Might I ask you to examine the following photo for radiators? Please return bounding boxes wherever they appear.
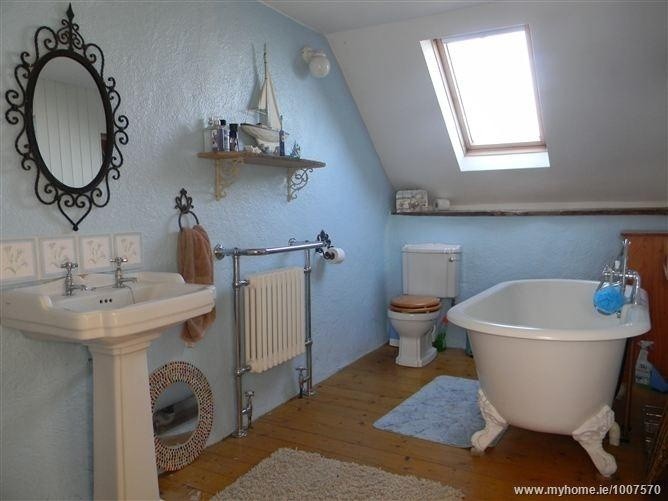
[246,267,303,372]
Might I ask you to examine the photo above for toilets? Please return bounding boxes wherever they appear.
[386,243,461,368]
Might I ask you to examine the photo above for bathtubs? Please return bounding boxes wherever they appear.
[447,279,651,476]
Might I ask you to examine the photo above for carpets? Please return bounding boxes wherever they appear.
[372,373,484,448]
[207,446,466,500]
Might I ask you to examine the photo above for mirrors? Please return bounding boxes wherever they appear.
[147,360,212,471]
[2,2,130,231]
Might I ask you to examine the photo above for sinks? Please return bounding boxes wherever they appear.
[1,269,217,347]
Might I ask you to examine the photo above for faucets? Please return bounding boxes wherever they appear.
[61,261,88,296]
[601,264,642,303]
[109,258,137,288]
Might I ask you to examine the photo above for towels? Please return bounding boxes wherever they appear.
[175,226,217,344]
